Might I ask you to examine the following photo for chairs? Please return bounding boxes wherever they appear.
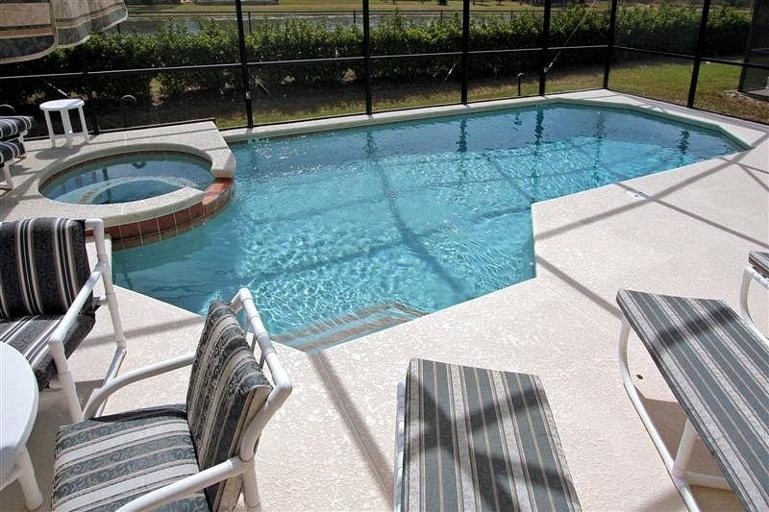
[0,215,129,424]
[0,104,34,191]
[49,286,293,512]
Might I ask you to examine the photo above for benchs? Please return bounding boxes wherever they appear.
[615,289,769,512]
[738,249,768,346]
[392,356,582,512]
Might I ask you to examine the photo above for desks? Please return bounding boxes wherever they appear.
[39,98,90,149]
[0,340,44,512]
